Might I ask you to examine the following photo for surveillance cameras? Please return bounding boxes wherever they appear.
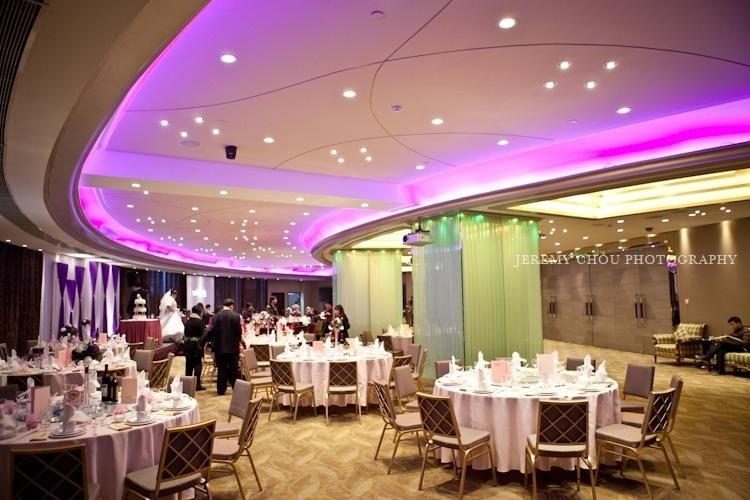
[225,145,237,159]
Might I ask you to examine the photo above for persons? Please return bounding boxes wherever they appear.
[184,304,207,391]
[158,288,185,344]
[695,316,750,376]
[265,296,278,322]
[242,302,256,324]
[285,300,350,346]
[203,304,215,325]
[125,279,149,318]
[198,299,242,395]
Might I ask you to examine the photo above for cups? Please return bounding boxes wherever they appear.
[386,323,413,336]
[449,356,603,394]
[284,346,387,361]
[251,314,307,328]
[248,329,296,344]
[147,313,159,319]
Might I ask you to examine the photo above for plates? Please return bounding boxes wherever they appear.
[2,329,124,373]
[1,388,192,439]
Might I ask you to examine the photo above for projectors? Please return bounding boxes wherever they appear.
[403,233,434,245]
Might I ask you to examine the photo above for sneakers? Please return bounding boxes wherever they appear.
[196,386,206,391]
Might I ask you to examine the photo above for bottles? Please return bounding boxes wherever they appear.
[99,361,118,403]
